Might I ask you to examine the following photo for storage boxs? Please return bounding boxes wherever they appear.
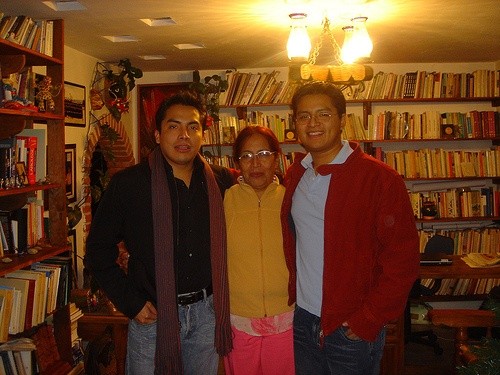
[68,290,93,313]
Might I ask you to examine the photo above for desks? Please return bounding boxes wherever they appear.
[381,254,500,375]
[77,309,130,375]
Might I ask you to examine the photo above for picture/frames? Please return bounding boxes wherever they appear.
[44,144,77,210]
[34,72,87,129]
[68,229,78,288]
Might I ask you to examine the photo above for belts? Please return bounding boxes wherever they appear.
[177,289,211,305]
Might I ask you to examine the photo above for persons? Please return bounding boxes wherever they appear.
[216,125,295,375]
[215,81,420,375]
[84,93,227,375]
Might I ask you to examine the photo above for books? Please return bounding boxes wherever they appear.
[342,110,499,137]
[2,62,36,105]
[201,112,295,145]
[360,69,499,97]
[360,142,500,178]
[206,148,294,175]
[0,257,85,375]
[417,226,500,269]
[5,123,48,185]
[415,277,500,296]
[411,299,483,324]
[0,12,54,57]
[225,70,296,106]
[0,200,43,262]
[408,183,500,218]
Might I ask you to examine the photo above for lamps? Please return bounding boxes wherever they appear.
[340,16,374,63]
[287,13,312,65]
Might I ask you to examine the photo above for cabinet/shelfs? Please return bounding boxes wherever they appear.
[201,97,500,301]
[0,17,77,375]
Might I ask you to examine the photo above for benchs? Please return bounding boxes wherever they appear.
[426,308,500,375]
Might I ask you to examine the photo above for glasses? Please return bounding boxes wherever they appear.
[238,150,275,161]
[295,111,337,125]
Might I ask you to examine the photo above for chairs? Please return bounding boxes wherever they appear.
[404,235,455,356]
